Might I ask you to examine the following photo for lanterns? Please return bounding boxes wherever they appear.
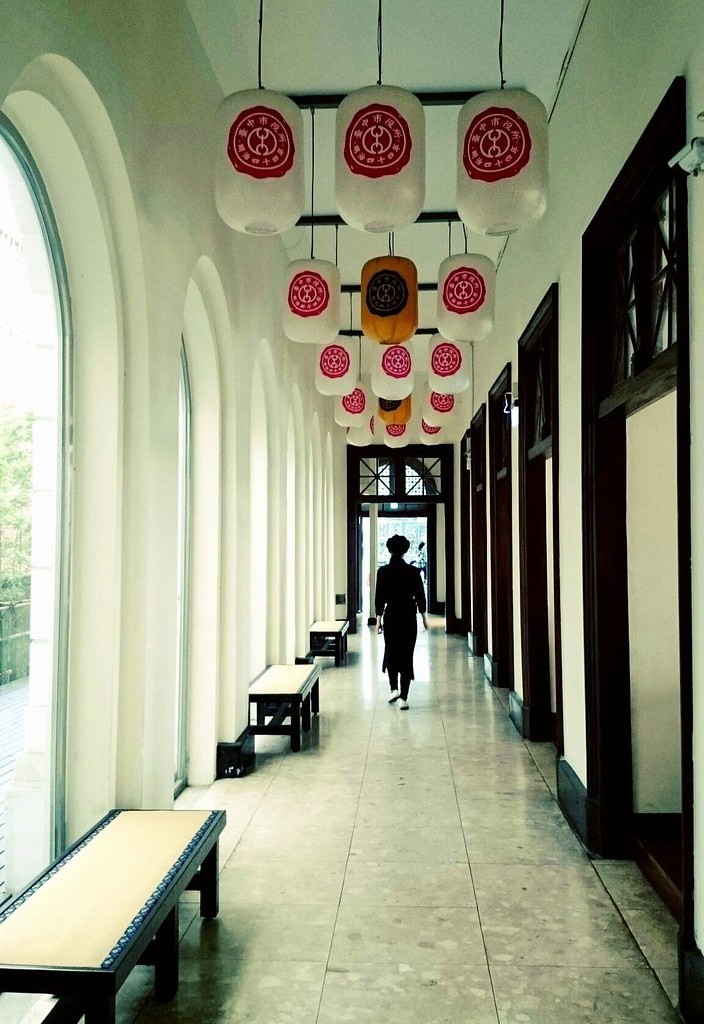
[372,340,413,400]
[360,255,418,345]
[437,253,496,342]
[429,334,470,394]
[315,335,356,395]
[455,88,550,237]
[282,259,341,344]
[334,85,426,234]
[212,89,304,236]
[333,381,456,448]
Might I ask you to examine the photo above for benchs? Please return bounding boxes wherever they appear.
[309,620,349,667]
[0,809,227,1024]
[249,664,322,751]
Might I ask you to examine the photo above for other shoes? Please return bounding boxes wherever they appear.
[397,698,412,710]
[387,689,401,703]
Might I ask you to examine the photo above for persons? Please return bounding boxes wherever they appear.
[416,542,427,583]
[375,534,428,710]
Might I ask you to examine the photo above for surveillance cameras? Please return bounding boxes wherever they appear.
[668,136,704,173]
